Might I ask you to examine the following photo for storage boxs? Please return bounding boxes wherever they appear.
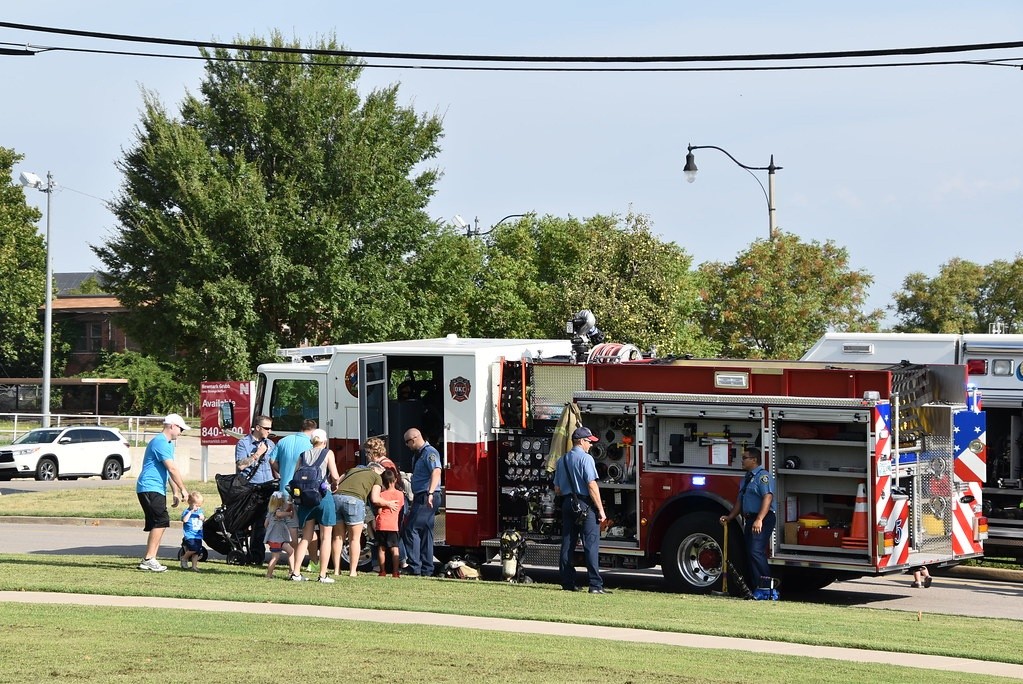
[785,522,797,545]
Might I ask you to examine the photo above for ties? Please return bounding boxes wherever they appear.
[739,474,753,526]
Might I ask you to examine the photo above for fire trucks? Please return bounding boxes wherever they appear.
[797,332,1023,572]
[251,308,988,599]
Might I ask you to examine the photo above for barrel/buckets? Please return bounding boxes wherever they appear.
[799,512,827,527]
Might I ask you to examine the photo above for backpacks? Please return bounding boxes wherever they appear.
[285,449,330,506]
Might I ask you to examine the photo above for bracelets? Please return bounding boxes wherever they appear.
[253,455,257,460]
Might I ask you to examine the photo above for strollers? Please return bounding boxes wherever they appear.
[178,473,256,565]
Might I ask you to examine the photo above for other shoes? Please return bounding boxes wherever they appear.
[393,571,400,578]
[911,581,921,588]
[180,556,188,569]
[378,571,386,576]
[924,576,932,588]
[401,566,420,576]
[191,567,199,572]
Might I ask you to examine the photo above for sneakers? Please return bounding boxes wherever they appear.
[317,575,335,582]
[140,557,167,572]
[291,573,310,581]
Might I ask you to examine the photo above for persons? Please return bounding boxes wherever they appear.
[331,462,400,577]
[911,565,932,588]
[404,427,442,576]
[375,469,405,578]
[268,419,319,573]
[552,426,613,595]
[286,429,340,583]
[366,503,379,546]
[136,413,190,572]
[719,447,777,600]
[263,491,295,581]
[235,416,280,566]
[181,491,205,572]
[364,437,409,571]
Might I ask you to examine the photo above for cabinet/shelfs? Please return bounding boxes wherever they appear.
[775,438,867,478]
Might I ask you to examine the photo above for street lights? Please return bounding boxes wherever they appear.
[682,145,776,246]
[20,172,52,428]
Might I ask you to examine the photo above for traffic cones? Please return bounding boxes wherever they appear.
[843,483,869,551]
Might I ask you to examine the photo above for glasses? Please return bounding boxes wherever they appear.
[405,437,417,444]
[256,423,272,430]
[179,427,183,433]
[742,456,754,460]
[583,438,592,444]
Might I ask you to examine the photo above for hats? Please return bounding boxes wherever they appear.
[311,429,327,441]
[163,414,191,430]
[572,427,599,441]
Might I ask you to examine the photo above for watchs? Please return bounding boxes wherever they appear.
[427,492,433,496]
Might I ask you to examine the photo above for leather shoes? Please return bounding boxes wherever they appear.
[562,587,588,593]
[588,589,613,594]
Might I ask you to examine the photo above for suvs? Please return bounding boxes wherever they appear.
[0,423,131,480]
